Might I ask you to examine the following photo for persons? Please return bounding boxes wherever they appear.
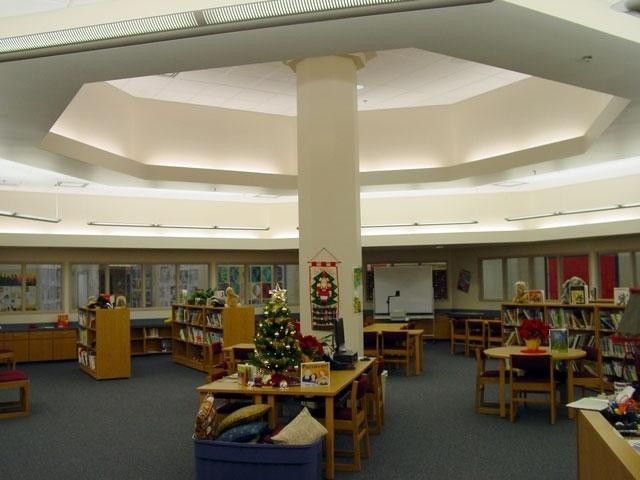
[302,368,329,386]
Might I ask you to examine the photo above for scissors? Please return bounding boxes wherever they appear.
[619,395,635,408]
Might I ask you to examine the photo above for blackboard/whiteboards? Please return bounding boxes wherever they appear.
[364,267,448,302]
[373,266,433,314]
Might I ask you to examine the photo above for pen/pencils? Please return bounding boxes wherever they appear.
[607,400,626,416]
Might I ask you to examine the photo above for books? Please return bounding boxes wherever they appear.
[76,308,96,373]
[501,286,639,394]
[171,307,224,345]
[300,361,331,389]
[549,328,569,354]
[564,397,610,411]
[237,364,261,387]
[149,326,171,352]
